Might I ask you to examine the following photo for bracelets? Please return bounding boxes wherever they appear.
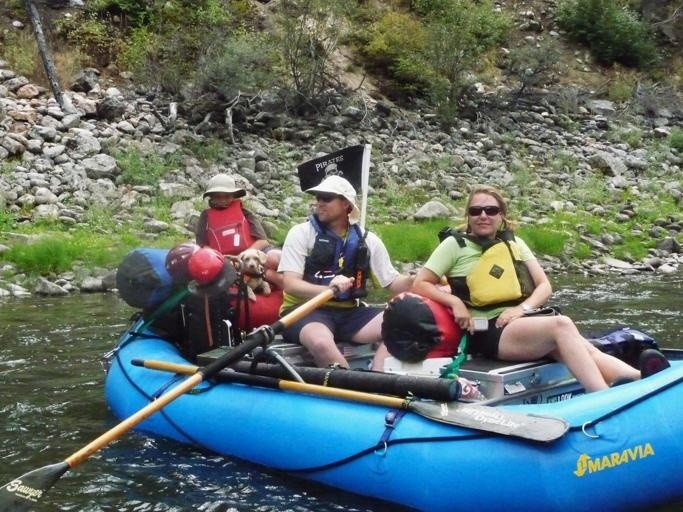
[520,302,531,312]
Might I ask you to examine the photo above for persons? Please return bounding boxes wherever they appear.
[411,184,670,394]
[277,175,417,373]
[196,173,284,289]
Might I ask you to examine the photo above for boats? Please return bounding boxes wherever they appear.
[101,312,683,512]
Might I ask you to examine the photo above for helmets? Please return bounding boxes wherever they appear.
[187,246,225,286]
[165,243,200,281]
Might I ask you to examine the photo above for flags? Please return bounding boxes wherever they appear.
[298,143,372,233]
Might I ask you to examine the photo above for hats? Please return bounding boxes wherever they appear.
[304,175,361,226]
[203,173,246,200]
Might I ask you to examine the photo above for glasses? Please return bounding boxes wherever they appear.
[316,192,345,202]
[468,206,502,216]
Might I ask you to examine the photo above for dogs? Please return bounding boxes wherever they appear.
[238,249,272,302]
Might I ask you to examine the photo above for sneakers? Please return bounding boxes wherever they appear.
[637,348,671,380]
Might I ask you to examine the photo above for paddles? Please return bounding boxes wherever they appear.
[127,357,569,448]
[0,270,355,506]
